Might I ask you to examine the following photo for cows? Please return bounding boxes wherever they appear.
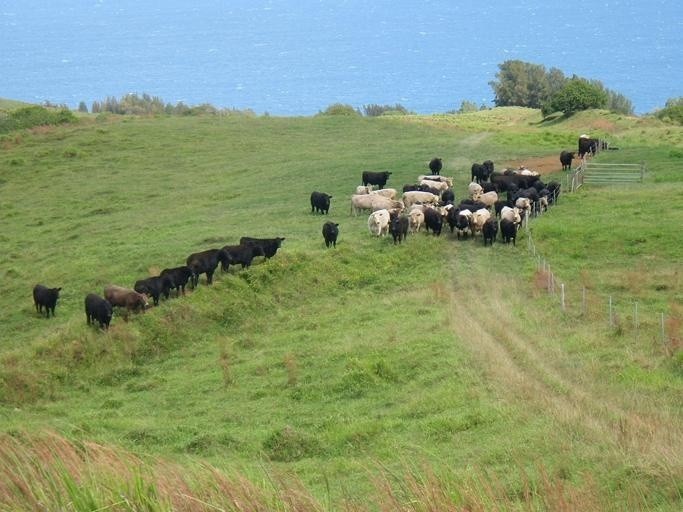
[32,284,62,319]
[310,191,332,215]
[347,157,562,247]
[322,220,340,249]
[557,132,604,170]
[84,235,286,329]
